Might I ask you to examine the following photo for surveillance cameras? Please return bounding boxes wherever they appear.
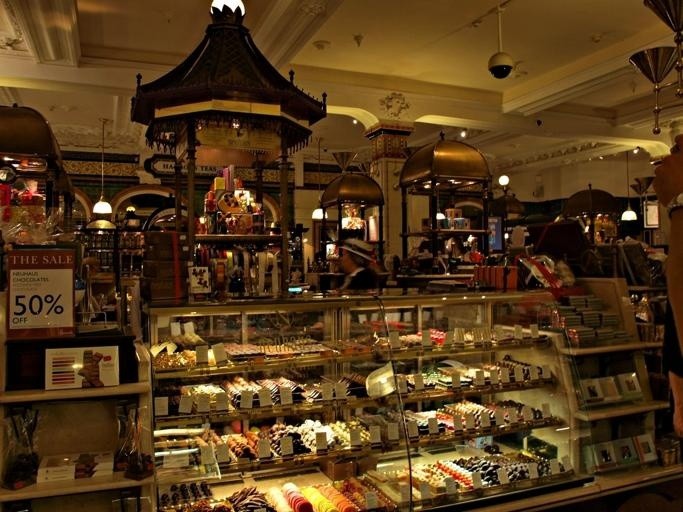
[488,53,516,80]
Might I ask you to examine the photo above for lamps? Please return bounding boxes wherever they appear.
[620,152,637,220]
[92,118,112,213]
[629,0,683,135]
[311,136,327,219]
[629,176,659,215]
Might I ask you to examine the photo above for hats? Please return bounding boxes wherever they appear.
[336,238,376,262]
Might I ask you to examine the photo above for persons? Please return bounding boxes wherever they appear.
[330,239,387,290]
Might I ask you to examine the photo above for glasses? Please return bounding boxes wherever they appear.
[338,251,350,258]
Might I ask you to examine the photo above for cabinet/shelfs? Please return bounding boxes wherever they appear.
[493,278,683,496]
[142,295,410,511]
[338,293,600,512]
[0,329,156,512]
